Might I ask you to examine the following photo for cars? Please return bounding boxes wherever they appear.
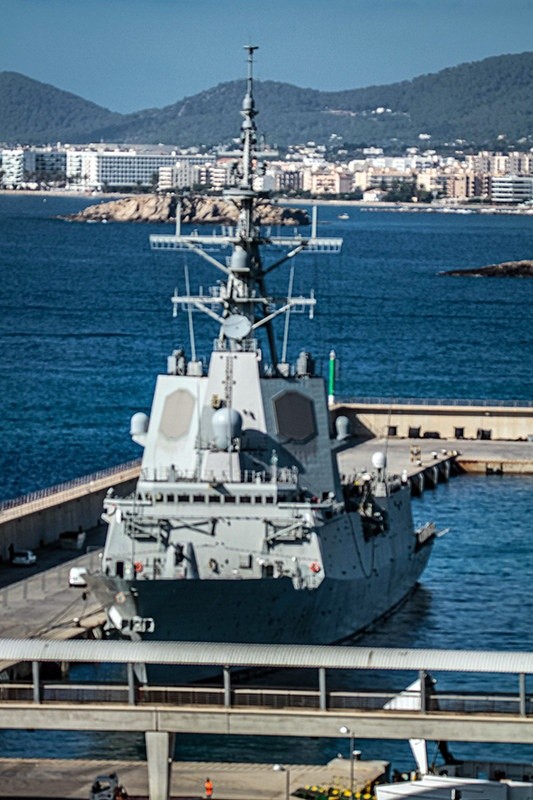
[11,550,38,567]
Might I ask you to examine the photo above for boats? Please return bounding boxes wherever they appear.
[66,46,436,685]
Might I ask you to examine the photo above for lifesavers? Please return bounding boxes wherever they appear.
[310,562,320,572]
[134,562,143,573]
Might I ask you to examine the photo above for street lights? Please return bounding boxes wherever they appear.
[273,763,290,800]
[338,725,354,799]
